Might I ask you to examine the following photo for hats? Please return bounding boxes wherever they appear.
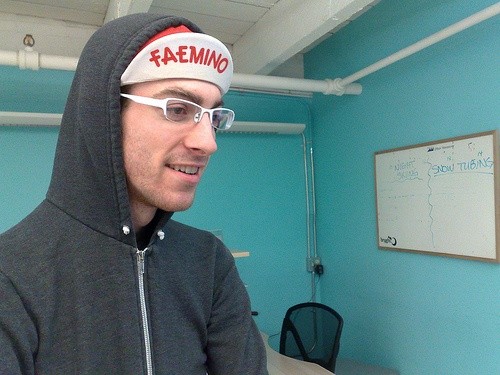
[119,25,233,96]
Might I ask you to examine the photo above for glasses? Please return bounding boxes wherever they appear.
[120,93,235,130]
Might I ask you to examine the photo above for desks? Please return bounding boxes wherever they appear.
[260,331,338,375]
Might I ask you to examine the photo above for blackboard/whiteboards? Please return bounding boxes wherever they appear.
[373,129,500,263]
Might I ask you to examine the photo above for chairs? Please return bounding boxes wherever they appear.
[278,302,345,374]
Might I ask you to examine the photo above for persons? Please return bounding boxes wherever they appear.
[0,13,271,375]
